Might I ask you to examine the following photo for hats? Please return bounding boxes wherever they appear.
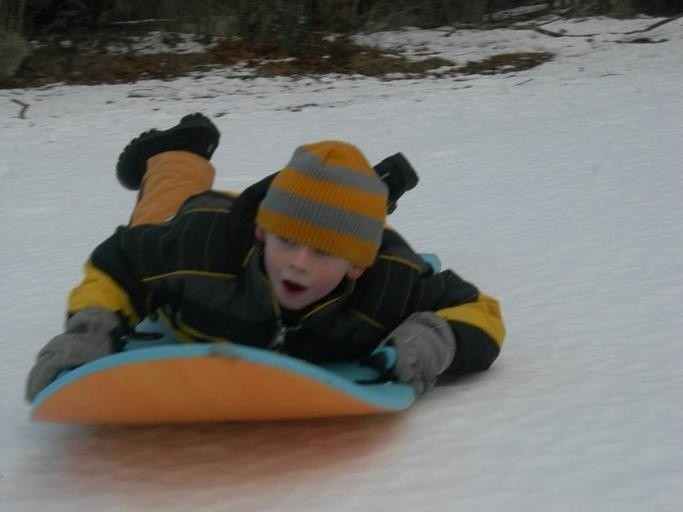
[254,139,389,268]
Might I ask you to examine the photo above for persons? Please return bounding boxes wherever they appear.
[26,112,506,404]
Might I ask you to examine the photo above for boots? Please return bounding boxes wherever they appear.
[115,113,221,191]
[373,153,419,215]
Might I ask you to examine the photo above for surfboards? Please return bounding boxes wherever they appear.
[29,253,442,423]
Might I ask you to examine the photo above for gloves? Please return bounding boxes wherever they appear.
[381,310,457,393]
[25,308,122,402]
